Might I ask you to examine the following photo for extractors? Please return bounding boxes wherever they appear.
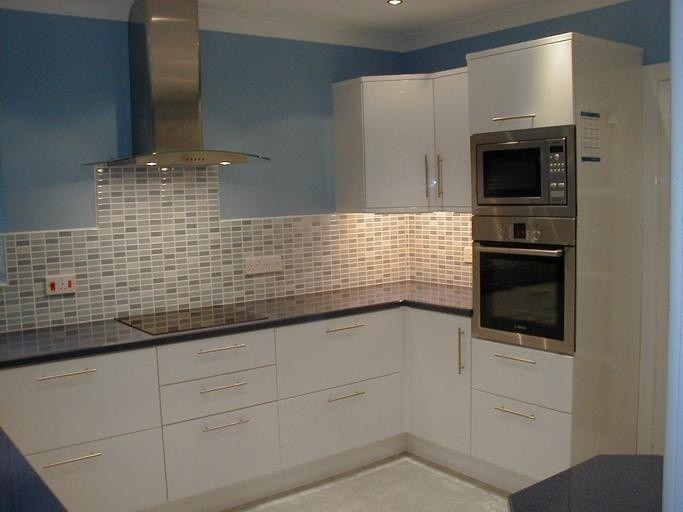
[80,0,272,168]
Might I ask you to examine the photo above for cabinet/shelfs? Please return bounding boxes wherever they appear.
[157,327,280,510]
[276,307,406,477]
[465,32,575,132]
[1,343,167,512]
[329,64,472,212]
[470,338,573,493]
[403,304,474,479]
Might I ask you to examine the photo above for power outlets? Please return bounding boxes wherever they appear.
[44,272,75,296]
[243,254,283,276]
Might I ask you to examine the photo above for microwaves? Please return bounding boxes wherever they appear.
[468,125,577,204]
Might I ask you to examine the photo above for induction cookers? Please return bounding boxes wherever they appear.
[114,304,268,336]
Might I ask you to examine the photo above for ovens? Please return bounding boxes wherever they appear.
[473,218,574,357]
